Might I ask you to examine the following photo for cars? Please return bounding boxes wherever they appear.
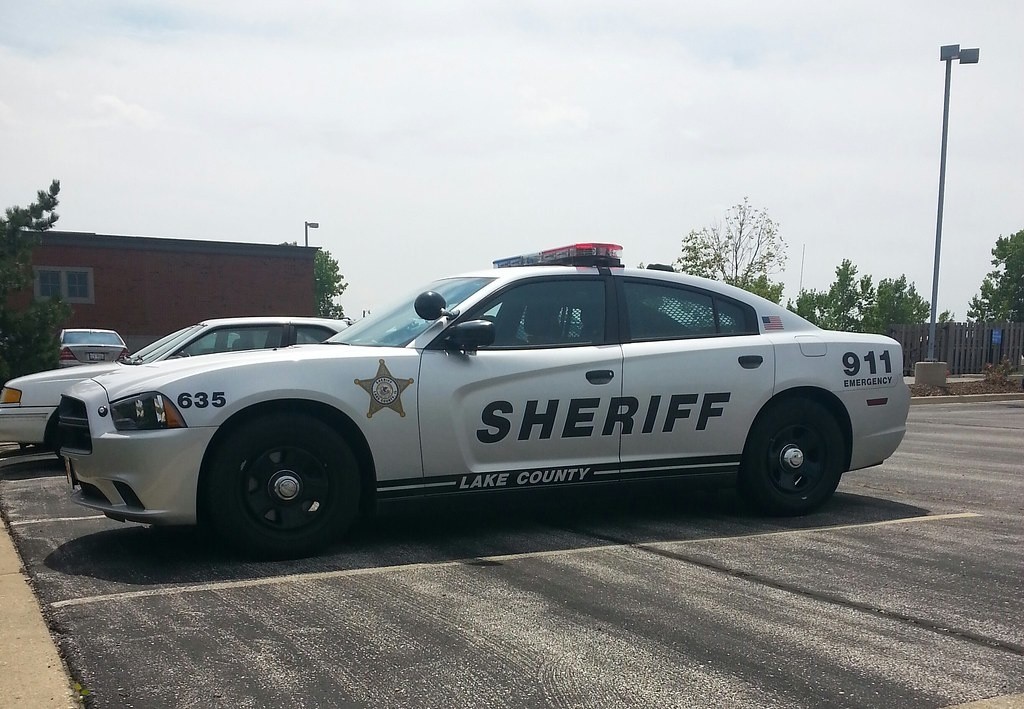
[0,317,349,468]
[59,243,911,563]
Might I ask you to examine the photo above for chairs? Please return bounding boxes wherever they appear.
[578,302,607,342]
[231,338,256,351]
[523,299,565,343]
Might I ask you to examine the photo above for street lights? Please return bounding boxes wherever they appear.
[305,221,319,247]
[913,44,979,382]
